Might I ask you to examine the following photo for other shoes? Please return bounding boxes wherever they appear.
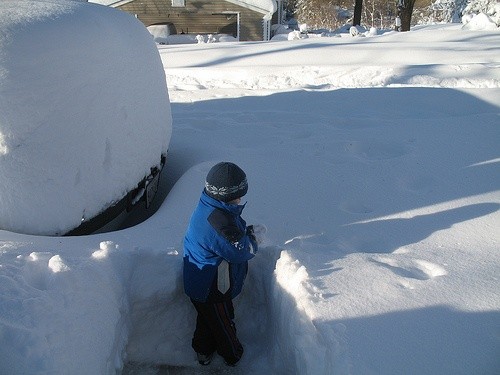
[196,352,211,365]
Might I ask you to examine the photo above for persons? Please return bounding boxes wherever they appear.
[182,162,267,367]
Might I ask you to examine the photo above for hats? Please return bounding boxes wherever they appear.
[204,162,248,201]
